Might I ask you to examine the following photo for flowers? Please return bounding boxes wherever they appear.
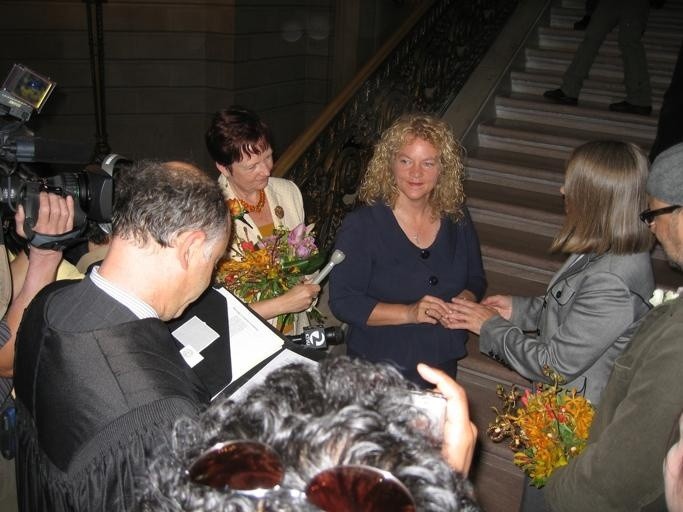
[217,222,317,327]
[486,369,598,487]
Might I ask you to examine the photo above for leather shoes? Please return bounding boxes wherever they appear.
[609,101,654,115]
[540,88,577,106]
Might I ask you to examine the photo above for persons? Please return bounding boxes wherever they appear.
[1,104,321,511]
[329,113,488,382]
[443,143,683,511]
[542,1,653,116]
[138,364,477,512]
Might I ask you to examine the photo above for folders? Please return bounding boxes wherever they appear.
[165,283,329,404]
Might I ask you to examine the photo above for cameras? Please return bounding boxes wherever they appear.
[407,388,449,440]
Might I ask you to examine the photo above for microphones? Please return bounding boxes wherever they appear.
[290,325,342,351]
[311,249,346,286]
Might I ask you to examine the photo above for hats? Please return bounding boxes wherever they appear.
[643,143,682,204]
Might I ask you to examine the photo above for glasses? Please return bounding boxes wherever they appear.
[181,438,417,512]
[633,204,683,224]
